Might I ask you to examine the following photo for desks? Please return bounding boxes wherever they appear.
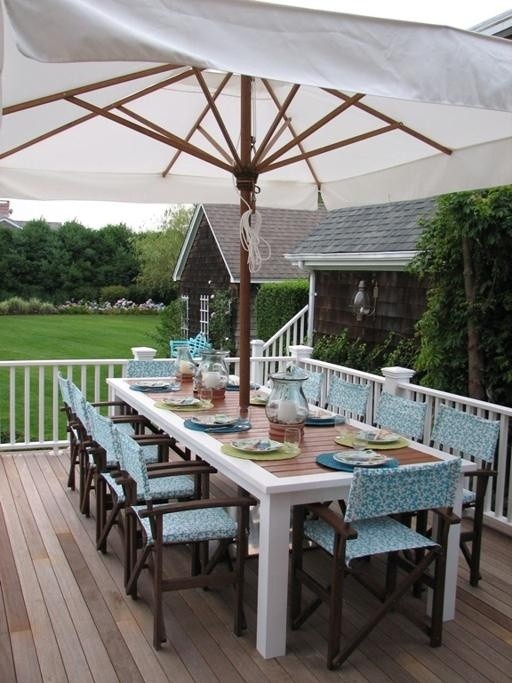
[103,374,478,658]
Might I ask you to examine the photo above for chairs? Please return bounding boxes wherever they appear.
[117,429,256,649]
[86,403,216,600]
[56,375,152,488]
[290,459,466,670]
[126,362,501,586]
[69,383,192,516]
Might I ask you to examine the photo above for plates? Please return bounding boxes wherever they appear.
[229,379,239,386]
[230,436,284,453]
[331,449,391,468]
[162,396,199,406]
[256,395,267,401]
[135,379,167,388]
[307,407,335,420]
[190,413,237,426]
[359,429,400,442]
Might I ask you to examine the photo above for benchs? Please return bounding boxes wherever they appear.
[170,335,213,358]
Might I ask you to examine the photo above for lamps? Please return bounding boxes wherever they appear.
[346,278,380,323]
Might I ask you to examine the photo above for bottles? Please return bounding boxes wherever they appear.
[174,346,197,375]
[264,371,309,424]
[192,349,231,390]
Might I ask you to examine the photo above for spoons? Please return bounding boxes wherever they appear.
[206,424,253,433]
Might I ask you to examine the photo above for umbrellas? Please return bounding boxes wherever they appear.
[1,2,512,430]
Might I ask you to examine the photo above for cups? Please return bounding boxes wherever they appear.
[283,427,303,454]
[237,405,254,427]
[170,372,182,391]
[200,387,213,405]
[334,415,347,442]
[349,429,369,450]
